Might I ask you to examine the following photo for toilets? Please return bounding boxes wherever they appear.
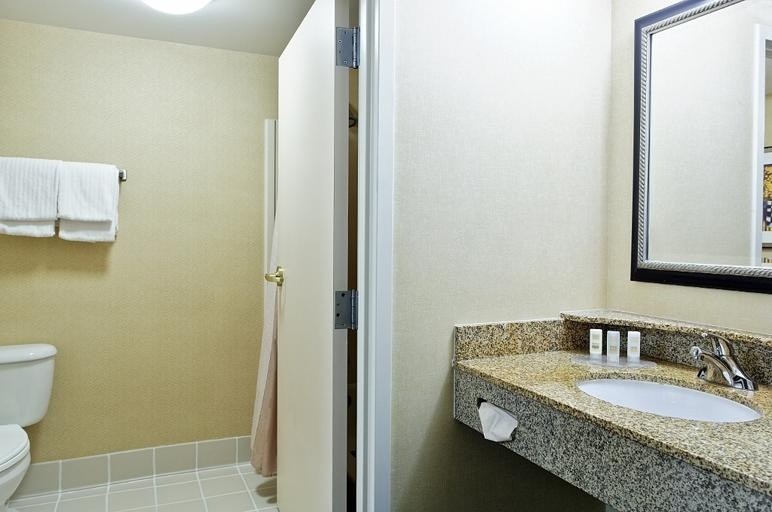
[0,345,56,511]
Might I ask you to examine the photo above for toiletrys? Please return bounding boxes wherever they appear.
[606,330,621,363]
[627,330,642,360]
[589,328,603,355]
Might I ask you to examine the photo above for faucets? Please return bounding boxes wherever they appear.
[690,332,758,390]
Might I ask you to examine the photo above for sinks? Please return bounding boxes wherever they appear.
[578,372,763,424]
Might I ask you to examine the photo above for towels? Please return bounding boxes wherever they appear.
[0,156,57,238]
[58,161,120,243]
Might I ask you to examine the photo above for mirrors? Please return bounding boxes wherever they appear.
[630,0,772,296]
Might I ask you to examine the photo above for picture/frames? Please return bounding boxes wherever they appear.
[760,146,772,245]
